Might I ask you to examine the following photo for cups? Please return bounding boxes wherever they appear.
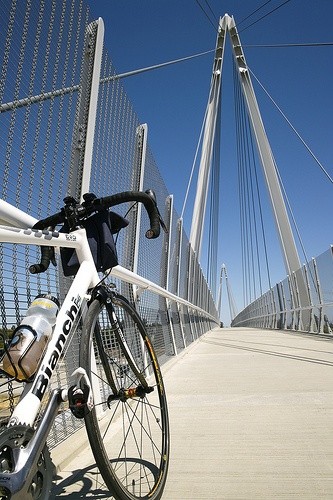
[3,292,60,382]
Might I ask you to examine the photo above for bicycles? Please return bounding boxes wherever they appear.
[1,191,173,500]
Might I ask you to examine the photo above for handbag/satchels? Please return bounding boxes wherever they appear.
[57,208,129,278]
